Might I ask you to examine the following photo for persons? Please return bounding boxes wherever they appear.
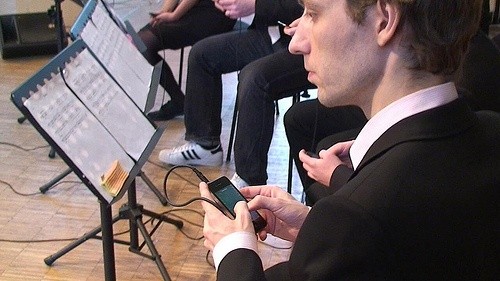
[158,0,324,197]
[284,91,419,208]
[131,0,238,120]
[198,0,500,280]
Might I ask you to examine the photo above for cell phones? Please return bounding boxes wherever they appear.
[306,151,320,159]
[208,175,267,233]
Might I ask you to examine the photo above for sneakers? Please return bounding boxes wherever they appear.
[231,172,250,191]
[147,100,185,121]
[159,139,223,165]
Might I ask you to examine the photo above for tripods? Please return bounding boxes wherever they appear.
[39,0,183,281]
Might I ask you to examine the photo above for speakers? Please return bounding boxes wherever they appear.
[0,11,68,59]
